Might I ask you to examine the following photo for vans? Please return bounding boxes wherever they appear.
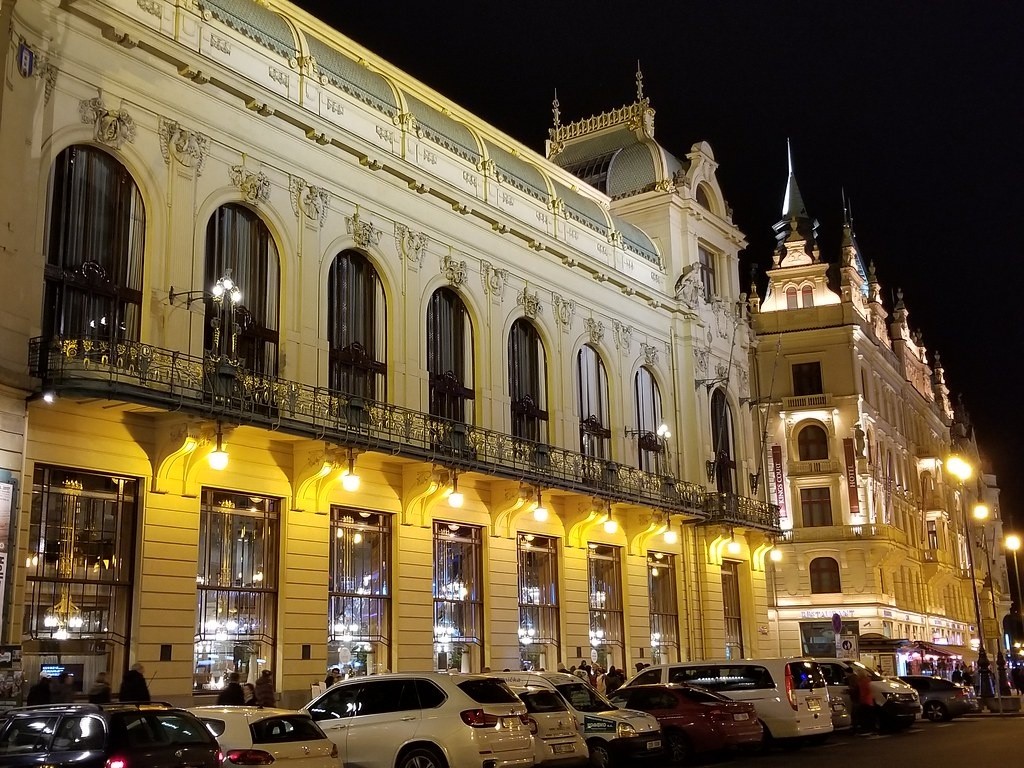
[816,658,924,735]
[459,673,662,768]
[608,657,833,752]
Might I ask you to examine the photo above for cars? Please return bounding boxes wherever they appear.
[127,704,343,768]
[604,684,764,760]
[828,697,852,734]
[884,675,980,723]
[509,685,589,768]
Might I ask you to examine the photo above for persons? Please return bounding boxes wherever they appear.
[482,660,650,694]
[844,667,879,737]
[952,662,1024,697]
[326,668,344,689]
[119,663,151,704]
[26,672,74,711]
[88,672,112,704]
[217,670,277,708]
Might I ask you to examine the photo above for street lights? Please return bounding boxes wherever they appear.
[946,456,996,698]
[1005,534,1024,620]
[972,505,1013,695]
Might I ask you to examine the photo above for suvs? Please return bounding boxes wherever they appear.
[274,669,537,768]
[0,700,224,768]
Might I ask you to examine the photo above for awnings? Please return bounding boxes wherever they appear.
[917,641,993,658]
